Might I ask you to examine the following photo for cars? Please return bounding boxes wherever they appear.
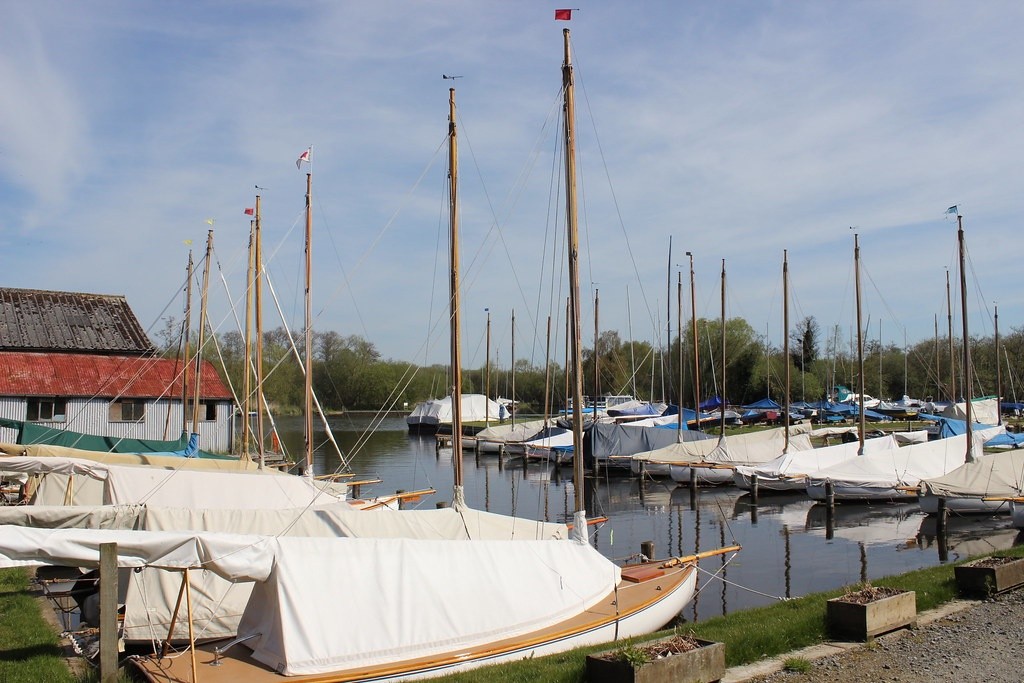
[851,395,883,407]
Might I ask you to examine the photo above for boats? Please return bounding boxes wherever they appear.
[558,396,649,415]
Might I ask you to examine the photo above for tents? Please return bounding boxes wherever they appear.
[699,395,907,423]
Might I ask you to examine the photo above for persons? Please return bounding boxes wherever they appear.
[924,396,937,427]
[499,404,504,424]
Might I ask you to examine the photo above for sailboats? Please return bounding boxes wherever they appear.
[918,306,1024,449]
[892,214,1024,530]
[0,28,742,683]
[608,272,812,476]
[731,490,818,598]
[867,319,948,418]
[462,288,742,467]
[804,233,983,500]
[643,257,815,484]
[709,249,900,491]
[668,482,749,615]
[804,501,928,583]
[896,515,1024,555]
